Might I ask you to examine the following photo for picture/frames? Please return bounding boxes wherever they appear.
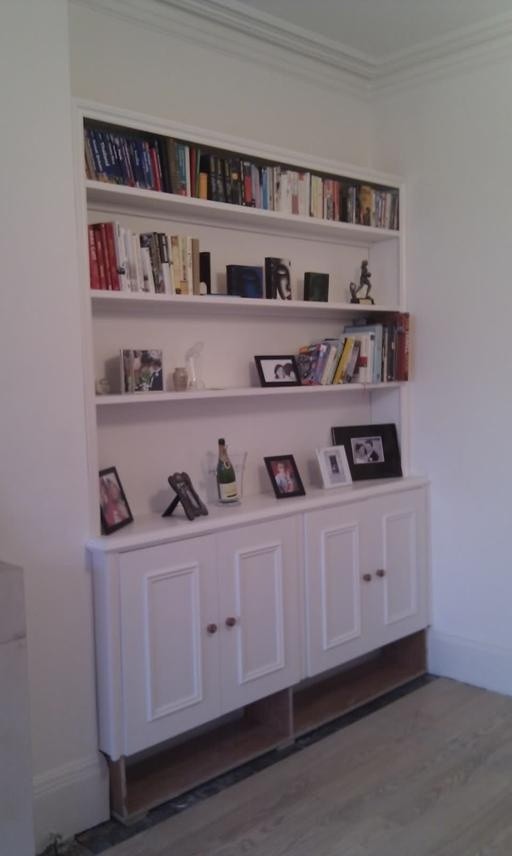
[254,355,300,385]
[162,472,209,521]
[264,454,306,498]
[315,445,353,489]
[99,466,134,535]
[331,423,402,480]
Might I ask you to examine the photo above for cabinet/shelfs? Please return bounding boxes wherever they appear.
[245,487,430,740]
[74,96,410,532]
[91,513,305,824]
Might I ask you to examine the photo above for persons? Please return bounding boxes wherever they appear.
[355,260,372,298]
[353,443,368,464]
[285,468,297,492]
[134,350,162,391]
[275,462,287,493]
[274,364,296,379]
[364,440,379,462]
[101,475,129,531]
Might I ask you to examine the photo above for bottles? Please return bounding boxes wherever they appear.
[216,436,240,504]
[174,366,188,393]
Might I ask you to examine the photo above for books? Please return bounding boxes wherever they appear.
[297,312,410,386]
[88,221,211,295]
[226,257,329,302]
[85,124,399,230]
